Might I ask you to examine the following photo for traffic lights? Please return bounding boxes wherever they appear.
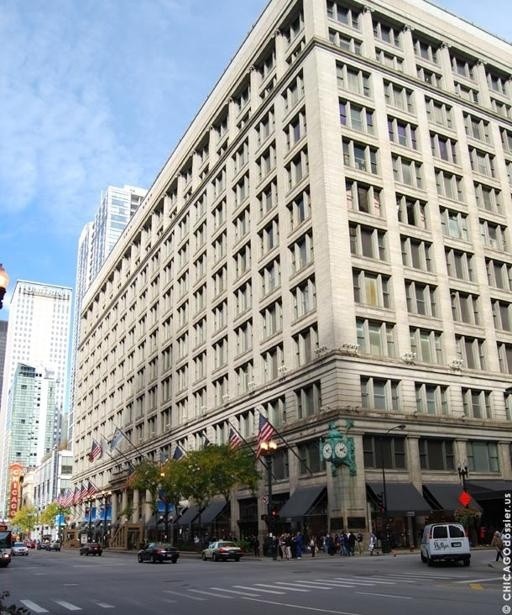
[261,510,280,522]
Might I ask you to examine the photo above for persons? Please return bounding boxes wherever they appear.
[491,530,503,561]
[479,525,490,547]
[368,531,377,555]
[309,536,316,557]
[193,532,233,544]
[269,532,304,560]
[253,536,260,557]
[32,539,40,549]
[323,530,363,556]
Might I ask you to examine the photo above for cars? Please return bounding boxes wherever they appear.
[80,543,102,556]
[0,539,60,569]
[137,542,179,564]
[201,541,242,561]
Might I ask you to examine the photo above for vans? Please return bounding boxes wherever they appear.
[420,522,471,567]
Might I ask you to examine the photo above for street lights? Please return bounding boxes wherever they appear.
[259,441,279,561]
[456,459,471,542]
[381,425,406,551]
[0,263,10,309]
[87,490,112,548]
[56,504,65,538]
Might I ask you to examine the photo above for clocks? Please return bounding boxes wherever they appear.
[321,441,349,461]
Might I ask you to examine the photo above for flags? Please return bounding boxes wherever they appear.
[88,426,127,463]
[159,413,275,465]
[56,482,96,505]
